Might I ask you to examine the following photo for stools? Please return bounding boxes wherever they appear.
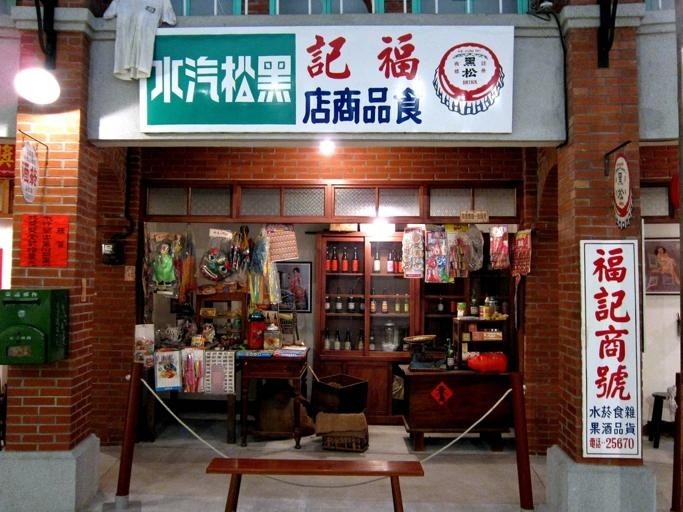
[646,391,676,450]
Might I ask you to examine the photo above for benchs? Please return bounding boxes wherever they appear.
[202,455,425,511]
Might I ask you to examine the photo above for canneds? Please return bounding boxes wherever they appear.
[479,306,489,319]
[457,302,467,317]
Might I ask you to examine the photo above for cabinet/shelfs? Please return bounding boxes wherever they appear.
[193,290,250,348]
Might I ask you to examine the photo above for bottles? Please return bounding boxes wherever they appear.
[383,323,395,347]
[322,326,376,352]
[469,296,508,318]
[373,248,381,273]
[437,297,444,314]
[446,339,454,371]
[246,310,266,349]
[325,288,409,313]
[262,322,283,350]
[386,248,403,274]
[325,245,359,273]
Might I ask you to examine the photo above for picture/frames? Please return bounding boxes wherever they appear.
[260,260,314,314]
[642,236,681,296]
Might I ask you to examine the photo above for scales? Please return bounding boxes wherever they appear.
[403,335,448,371]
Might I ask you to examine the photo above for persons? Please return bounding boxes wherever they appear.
[652,244,680,286]
[287,265,306,307]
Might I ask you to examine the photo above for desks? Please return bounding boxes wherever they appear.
[131,336,263,444]
[232,342,316,450]
